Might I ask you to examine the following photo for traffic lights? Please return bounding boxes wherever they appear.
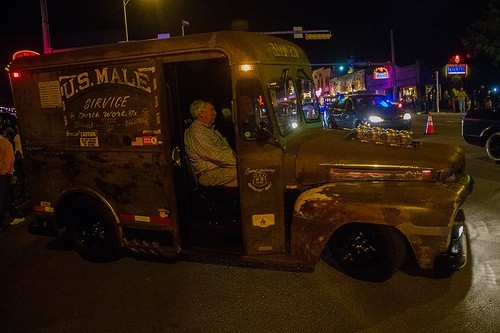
[338,65,344,71]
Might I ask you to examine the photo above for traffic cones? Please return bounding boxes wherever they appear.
[423,111,438,135]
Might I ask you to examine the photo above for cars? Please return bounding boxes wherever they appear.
[277,100,336,122]
[462,106,500,163]
[322,90,412,131]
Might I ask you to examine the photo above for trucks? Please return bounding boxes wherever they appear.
[9,31,476,281]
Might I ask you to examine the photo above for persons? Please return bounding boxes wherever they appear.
[238,96,270,138]
[184,99,239,187]
[0,127,25,225]
[411,85,491,112]
